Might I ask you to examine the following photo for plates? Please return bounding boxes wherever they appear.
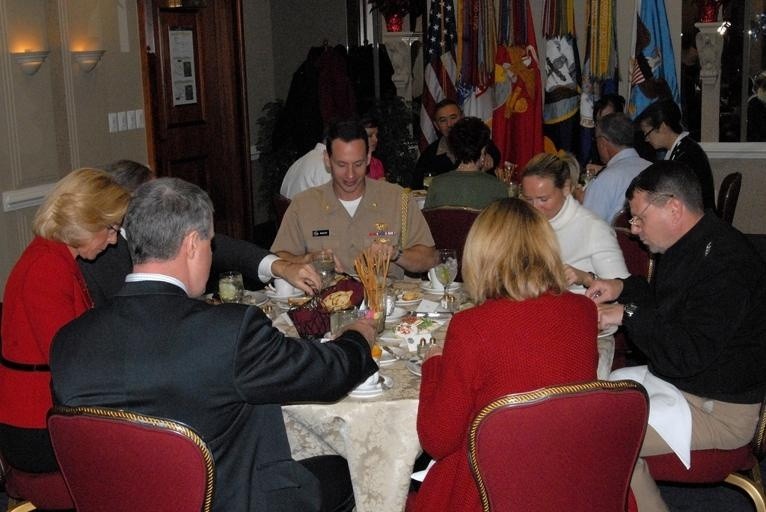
[597,322,620,339]
[219,248,466,400]
[196,290,270,308]
[411,190,428,199]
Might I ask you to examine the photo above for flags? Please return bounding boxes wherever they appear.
[414,1,680,181]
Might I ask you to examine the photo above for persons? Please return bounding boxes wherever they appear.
[415,95,716,292]
[0,160,377,510]
[268,117,438,280]
[581,163,765,512]
[402,197,637,512]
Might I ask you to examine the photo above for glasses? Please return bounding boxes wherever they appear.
[626,192,679,229]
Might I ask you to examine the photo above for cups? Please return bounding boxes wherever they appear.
[582,168,595,183]
[423,172,435,192]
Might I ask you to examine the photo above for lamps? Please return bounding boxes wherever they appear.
[71,49,106,74]
[10,50,50,76]
